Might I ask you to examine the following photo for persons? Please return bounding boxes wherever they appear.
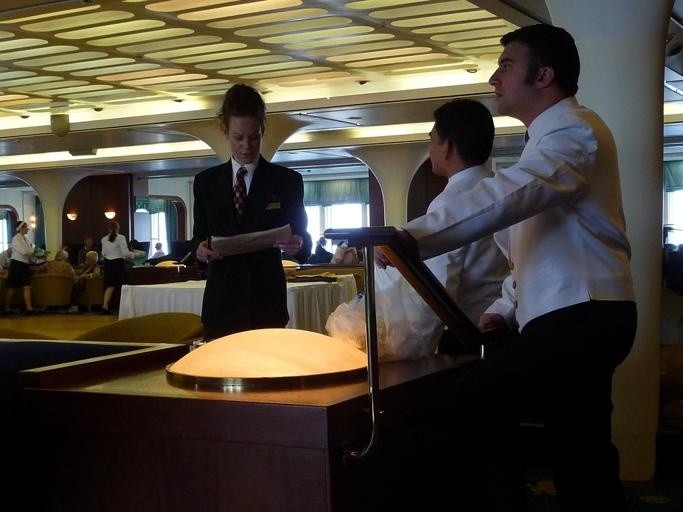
[188,84,313,341]
[312,235,333,264]
[2,220,167,315]
[407,97,510,356]
[372,25,638,510]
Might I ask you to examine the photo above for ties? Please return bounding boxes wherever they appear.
[233,167,247,212]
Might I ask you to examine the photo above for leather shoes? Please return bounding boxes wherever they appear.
[25,309,40,315]
[4,310,18,315]
[101,307,109,315]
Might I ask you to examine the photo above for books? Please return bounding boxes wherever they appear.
[207,223,294,261]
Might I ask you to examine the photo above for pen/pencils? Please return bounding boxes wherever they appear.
[207,232,211,265]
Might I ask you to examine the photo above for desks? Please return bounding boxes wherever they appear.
[115,273,355,338]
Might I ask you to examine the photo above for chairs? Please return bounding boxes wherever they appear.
[29,272,103,313]
